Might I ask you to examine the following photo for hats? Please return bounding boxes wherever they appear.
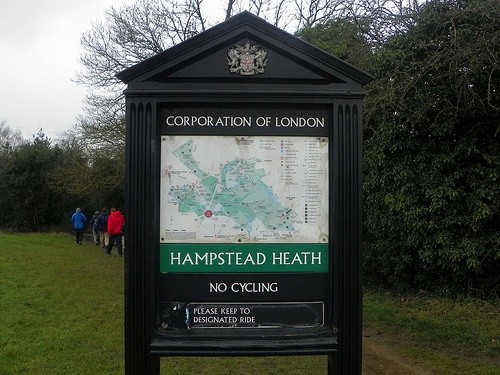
[75,207,107,217]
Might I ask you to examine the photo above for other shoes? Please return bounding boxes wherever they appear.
[77,239,123,257]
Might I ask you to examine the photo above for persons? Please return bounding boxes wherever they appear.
[93,208,109,247]
[107,208,124,256]
[71,208,87,245]
[91,211,101,245]
